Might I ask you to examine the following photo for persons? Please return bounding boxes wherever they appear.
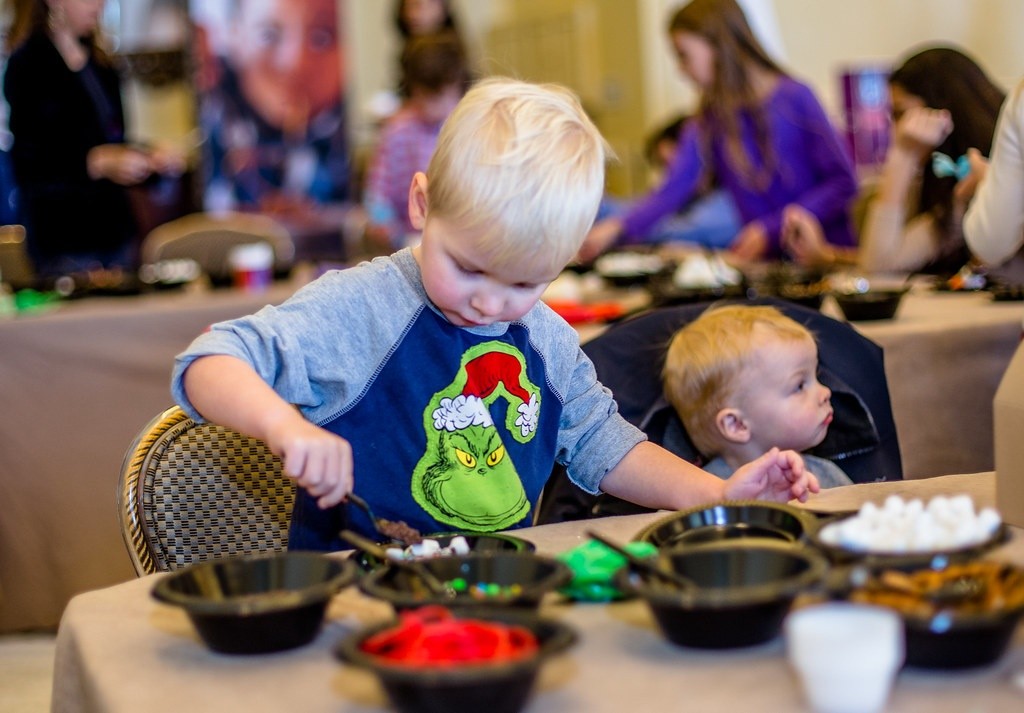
[582,0,1024,278]
[664,305,854,494]
[364,0,468,248]
[3,0,185,287]
[171,74,819,552]
[189,0,349,206]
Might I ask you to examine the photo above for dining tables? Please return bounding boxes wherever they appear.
[0,241,1024,713]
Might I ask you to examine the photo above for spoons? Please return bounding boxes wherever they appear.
[345,490,422,544]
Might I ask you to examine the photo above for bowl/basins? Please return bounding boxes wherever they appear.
[334,610,580,713]
[357,549,573,620]
[150,552,360,655]
[812,519,1015,571]
[630,501,822,547]
[824,558,1024,670]
[549,242,908,322]
[613,539,832,651]
[348,533,536,590]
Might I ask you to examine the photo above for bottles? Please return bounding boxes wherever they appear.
[993,335,1023,529]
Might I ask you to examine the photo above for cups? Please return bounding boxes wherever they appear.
[784,606,904,713]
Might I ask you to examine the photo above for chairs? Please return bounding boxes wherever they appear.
[538,303,905,525]
[141,211,295,273]
[115,405,299,578]
